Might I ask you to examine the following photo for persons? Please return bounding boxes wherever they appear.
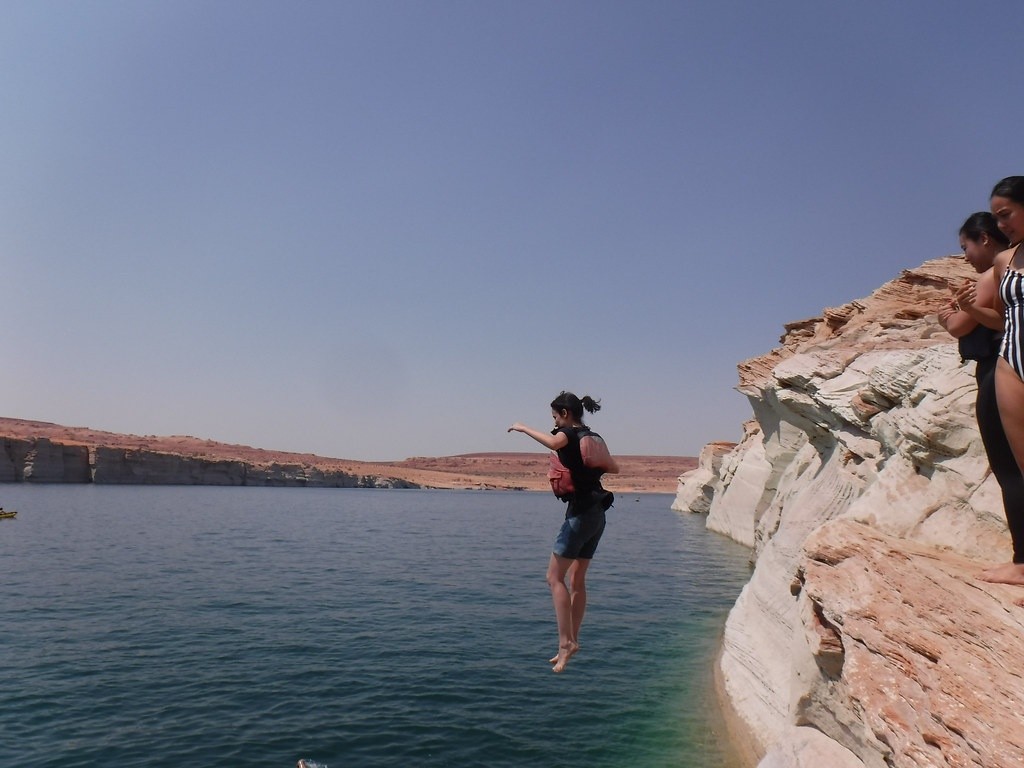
[938,212,1024,584]
[955,176,1024,609]
[508,391,614,673]
